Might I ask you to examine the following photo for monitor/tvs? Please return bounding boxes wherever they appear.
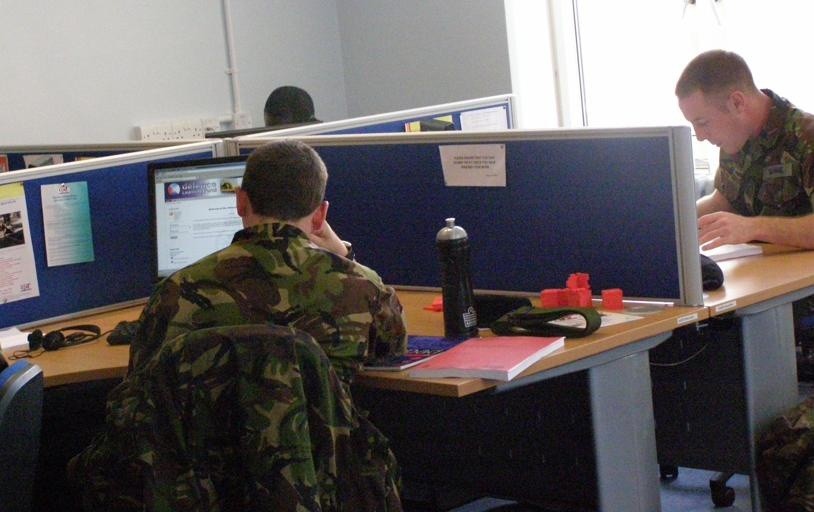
[420,116,454,129]
[147,154,247,283]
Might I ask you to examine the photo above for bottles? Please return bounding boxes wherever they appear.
[435,216,478,339]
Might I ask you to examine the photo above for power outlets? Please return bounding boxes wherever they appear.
[134,116,222,145]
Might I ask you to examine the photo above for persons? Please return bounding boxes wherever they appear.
[255,84,318,127]
[123,137,408,512]
[674,47,813,254]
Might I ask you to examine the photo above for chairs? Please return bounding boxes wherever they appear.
[0,349,53,510]
[61,321,405,511]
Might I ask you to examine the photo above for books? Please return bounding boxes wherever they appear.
[694,239,764,262]
[410,335,568,380]
[364,332,468,369]
[0,326,47,356]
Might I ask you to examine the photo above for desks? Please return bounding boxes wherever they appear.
[0,268,708,511]
[652,221,813,511]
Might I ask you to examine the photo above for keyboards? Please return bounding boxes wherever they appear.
[106,319,138,346]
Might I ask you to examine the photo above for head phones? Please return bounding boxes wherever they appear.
[28,325,102,350]
